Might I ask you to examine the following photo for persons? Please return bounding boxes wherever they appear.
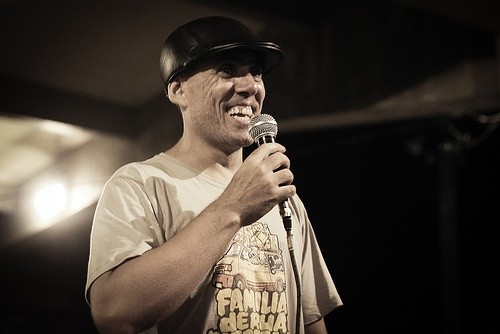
[85,16,343,334]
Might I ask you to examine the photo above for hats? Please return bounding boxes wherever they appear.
[158,16,286,94]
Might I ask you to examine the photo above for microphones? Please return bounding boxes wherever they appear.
[248,114,292,237]
